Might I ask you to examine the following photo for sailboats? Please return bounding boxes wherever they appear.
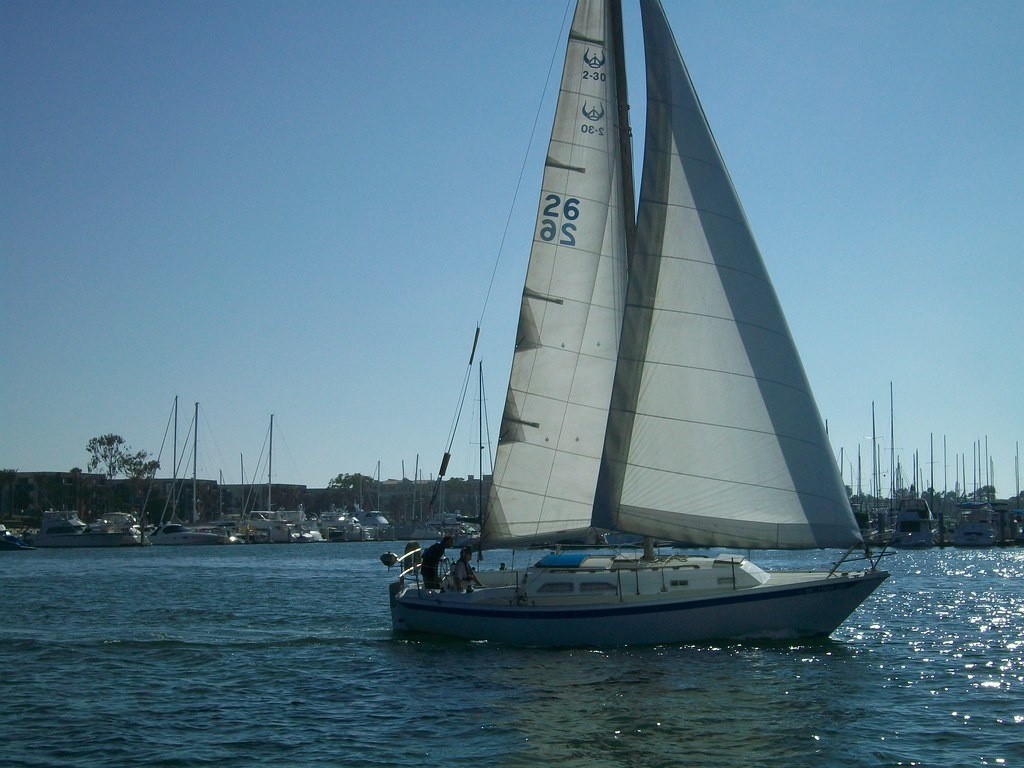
[375,0,901,644]
[815,379,1023,549]
[0,361,495,552]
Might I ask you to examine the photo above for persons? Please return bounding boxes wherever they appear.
[453,549,483,588]
[421,536,454,588]
